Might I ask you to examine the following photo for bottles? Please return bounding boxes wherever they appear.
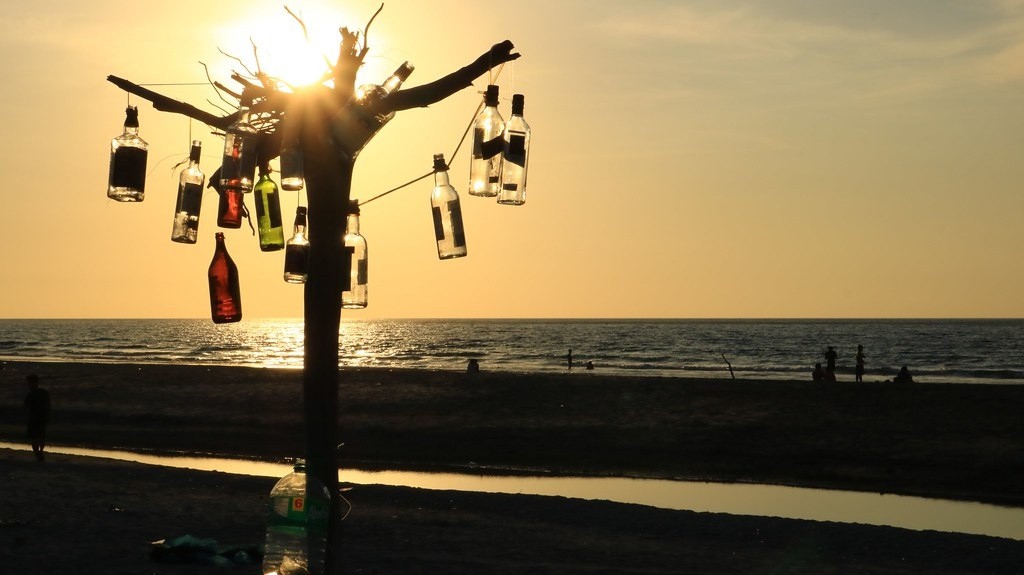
[220,98,257,192]
[469,85,505,197]
[107,106,150,202]
[254,162,284,251]
[497,94,531,205]
[340,199,368,308]
[209,233,242,323]
[314,61,416,164]
[284,206,310,284]
[279,116,303,191]
[172,140,205,244]
[430,153,467,260]
[262,465,330,574]
[217,139,243,229]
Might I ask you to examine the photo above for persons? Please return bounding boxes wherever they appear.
[469,360,480,370]
[587,361,593,369]
[825,346,837,382]
[855,344,865,382]
[894,366,913,384]
[813,365,826,384]
[568,349,572,369]
[23,375,50,461]
